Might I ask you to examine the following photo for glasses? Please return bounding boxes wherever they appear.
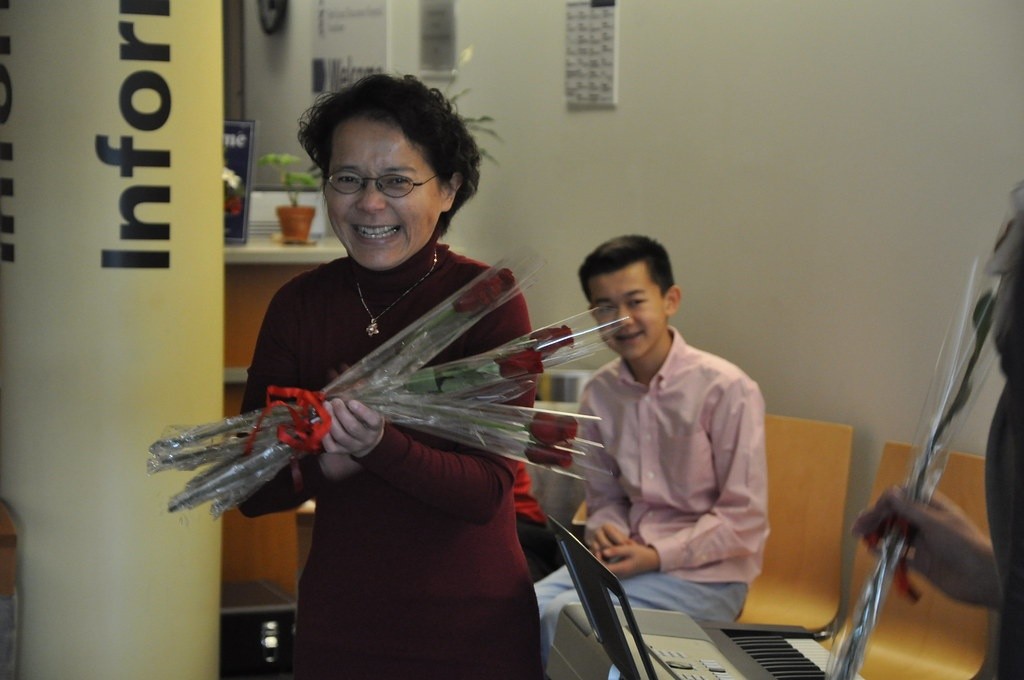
[323,169,439,199]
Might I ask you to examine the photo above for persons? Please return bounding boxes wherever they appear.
[857,207,1024,680]
[234,72,546,680]
[531,235,771,670]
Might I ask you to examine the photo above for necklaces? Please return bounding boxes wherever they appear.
[356,248,438,337]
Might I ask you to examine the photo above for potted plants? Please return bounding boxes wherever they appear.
[261,153,316,242]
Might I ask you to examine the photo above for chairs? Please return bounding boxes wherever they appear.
[756,414,994,680]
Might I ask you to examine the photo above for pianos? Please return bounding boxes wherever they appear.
[527,511,870,679]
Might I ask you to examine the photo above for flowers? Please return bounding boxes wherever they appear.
[146,256,633,516]
